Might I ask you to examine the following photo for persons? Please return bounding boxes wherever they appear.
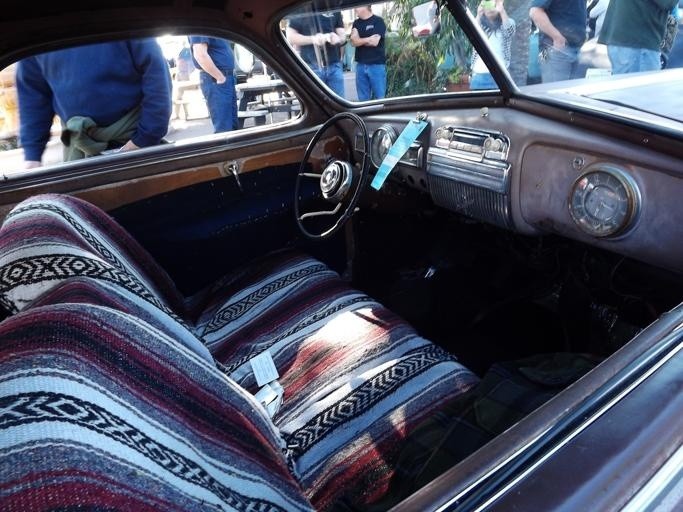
[467,1,517,91]
[529,0,587,84]
[349,5,389,102]
[285,11,348,103]
[598,0,680,74]
[190,33,237,133]
[15,29,174,169]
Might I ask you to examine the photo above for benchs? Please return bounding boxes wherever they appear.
[238,96,297,128]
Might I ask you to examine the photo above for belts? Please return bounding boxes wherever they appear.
[222,71,233,76]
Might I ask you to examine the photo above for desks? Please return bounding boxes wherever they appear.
[235,79,299,130]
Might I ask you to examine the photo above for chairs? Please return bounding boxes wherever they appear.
[1,191,384,381]
[0,303,481,512]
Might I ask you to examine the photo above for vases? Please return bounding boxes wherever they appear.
[448,74,470,92]
